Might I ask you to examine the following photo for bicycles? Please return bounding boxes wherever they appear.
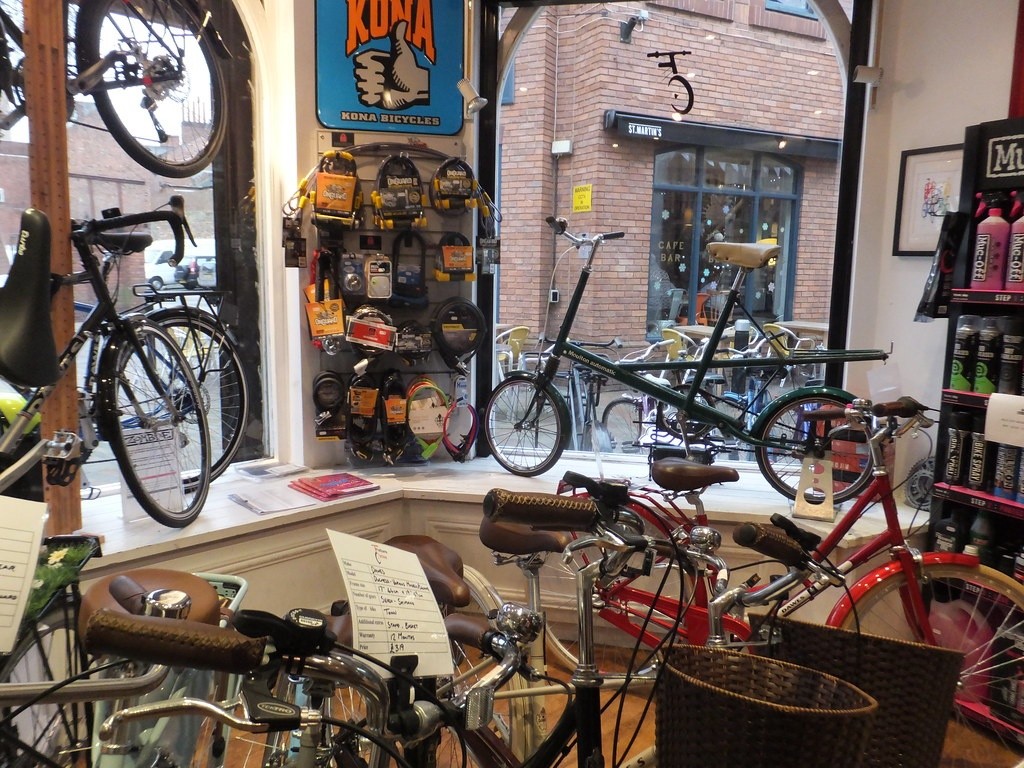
[482,213,890,501]
[0,197,215,530]
[484,324,822,455]
[72,234,251,490]
[0,0,235,177]
[4,487,965,768]
[526,396,1024,767]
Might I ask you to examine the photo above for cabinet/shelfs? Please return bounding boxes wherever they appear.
[923,118,1024,742]
[315,129,471,449]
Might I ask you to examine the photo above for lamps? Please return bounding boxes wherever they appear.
[459,76,487,116]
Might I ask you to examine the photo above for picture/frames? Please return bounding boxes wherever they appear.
[892,144,967,256]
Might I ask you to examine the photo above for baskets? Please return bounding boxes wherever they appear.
[747,612,968,768]
[655,643,879,768]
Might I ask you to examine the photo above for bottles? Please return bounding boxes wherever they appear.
[932,190,1024,583]
